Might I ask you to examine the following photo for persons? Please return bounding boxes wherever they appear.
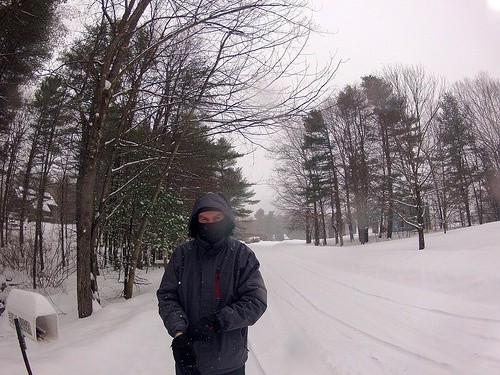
[155,190,268,375]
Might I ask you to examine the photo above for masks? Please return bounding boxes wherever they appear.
[197,216,232,245]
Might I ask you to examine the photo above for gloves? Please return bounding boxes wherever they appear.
[172,334,197,373]
[186,312,221,341]
